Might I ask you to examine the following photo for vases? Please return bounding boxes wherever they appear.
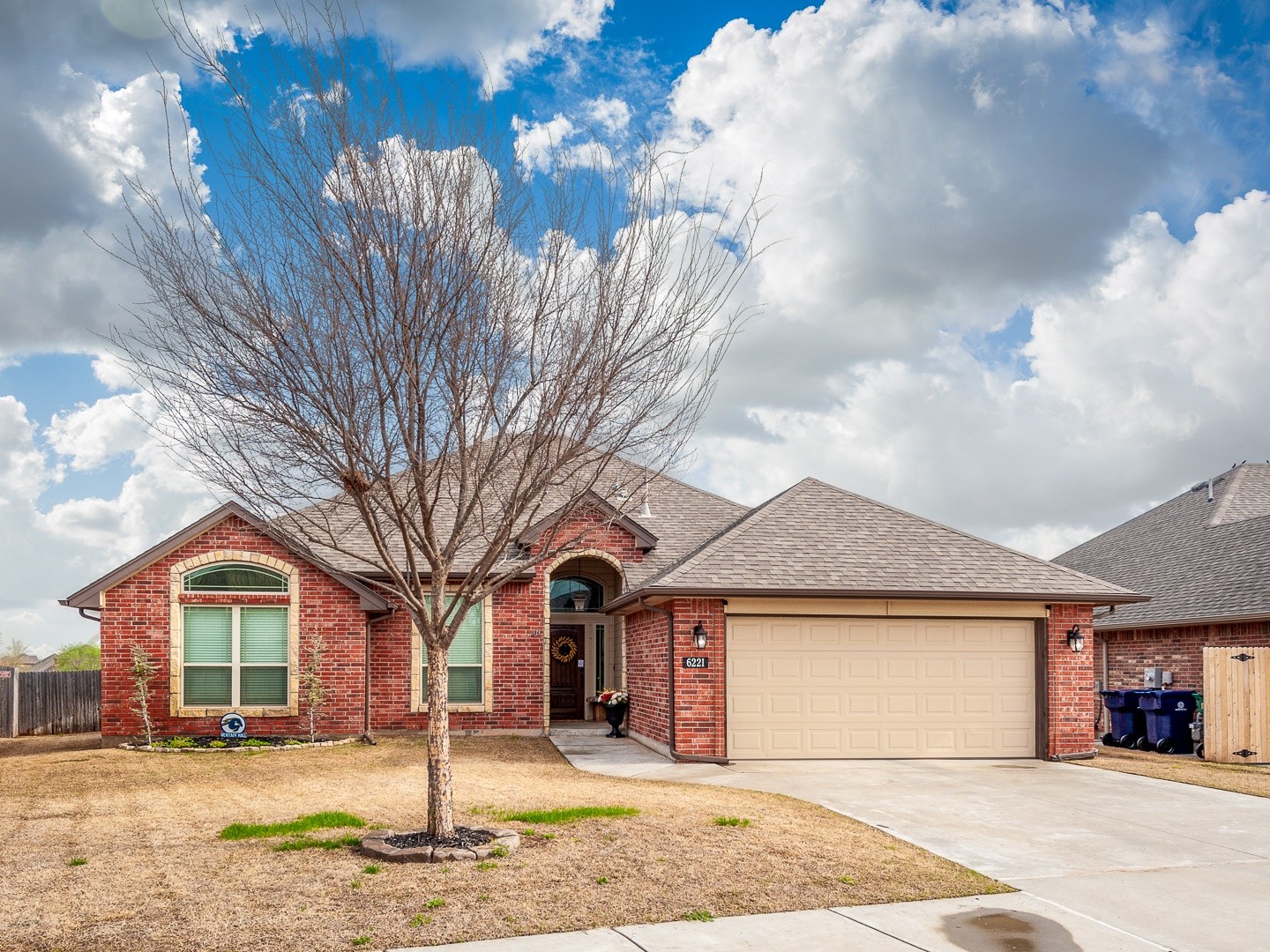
[604,704,626,739]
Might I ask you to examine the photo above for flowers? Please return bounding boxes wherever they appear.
[596,686,629,706]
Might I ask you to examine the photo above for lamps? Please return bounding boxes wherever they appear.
[692,620,708,650]
[1066,625,1085,653]
[573,557,587,611]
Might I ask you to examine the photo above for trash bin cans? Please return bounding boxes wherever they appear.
[1134,689,1197,753]
[1099,688,1153,747]
